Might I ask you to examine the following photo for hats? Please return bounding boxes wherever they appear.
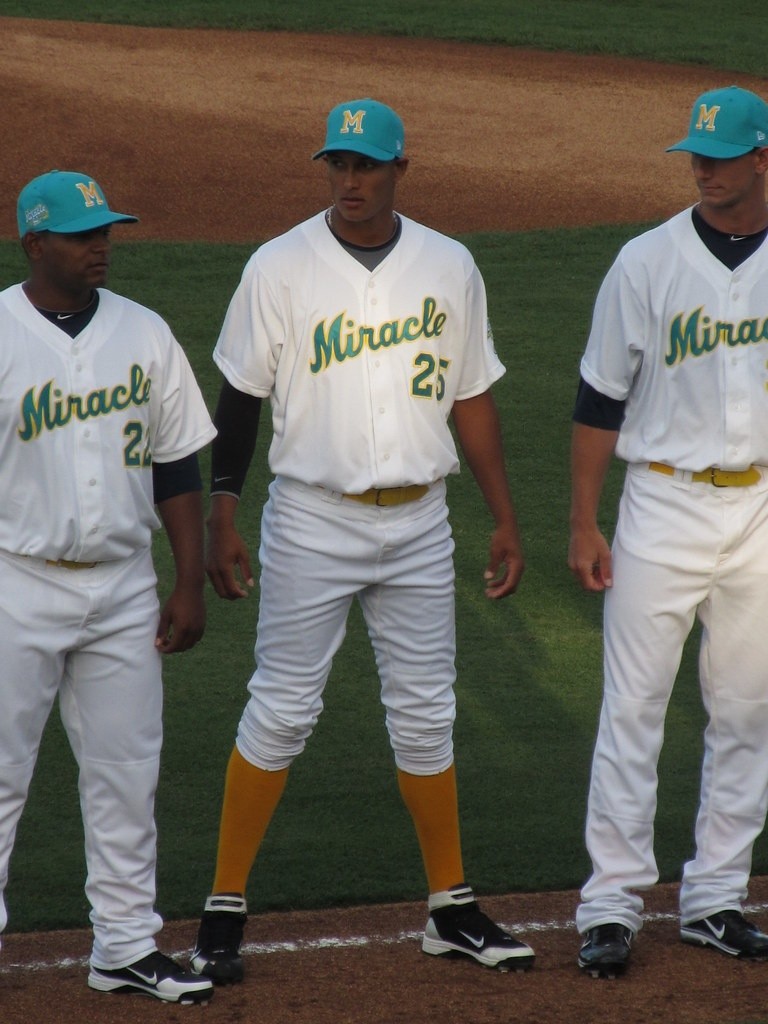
[314,95,405,167]
[665,85,768,159]
[18,173,136,237]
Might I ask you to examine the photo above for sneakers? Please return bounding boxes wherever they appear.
[679,912,767,960]
[577,923,634,978]
[89,950,215,1000]
[422,887,534,973]
[188,895,248,986]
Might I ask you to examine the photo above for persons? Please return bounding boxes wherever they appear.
[0,168,219,1006]
[565,85,768,974]
[187,98,526,985]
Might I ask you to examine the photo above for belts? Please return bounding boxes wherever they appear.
[343,484,430,510]
[43,559,98,572]
[649,461,762,488]
